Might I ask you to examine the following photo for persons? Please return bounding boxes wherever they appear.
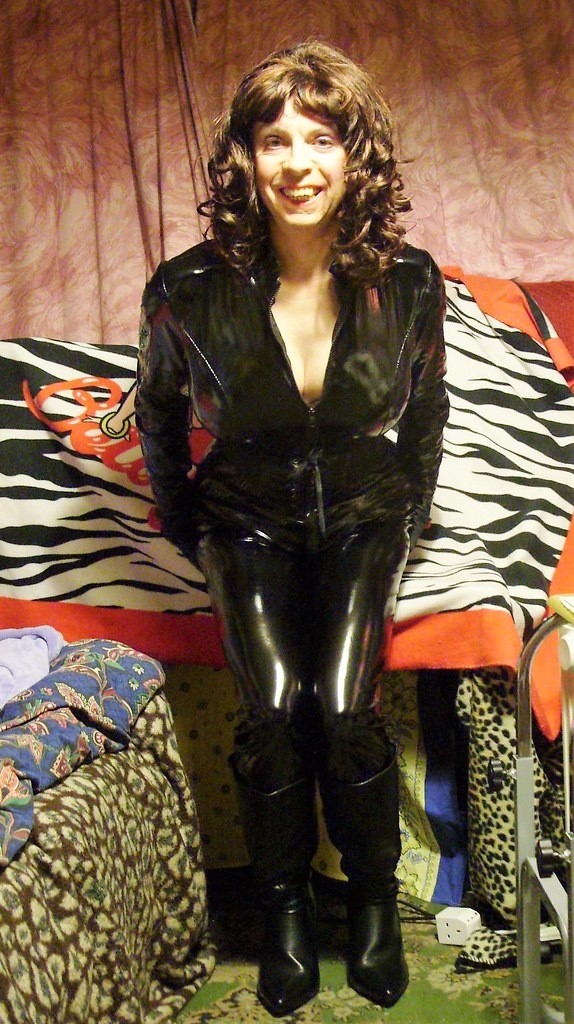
[134,41,451,1018]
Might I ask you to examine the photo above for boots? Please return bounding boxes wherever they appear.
[224,743,320,1017]
[315,748,409,1007]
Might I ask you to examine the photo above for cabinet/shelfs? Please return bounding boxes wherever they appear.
[515,594,574,1024]
